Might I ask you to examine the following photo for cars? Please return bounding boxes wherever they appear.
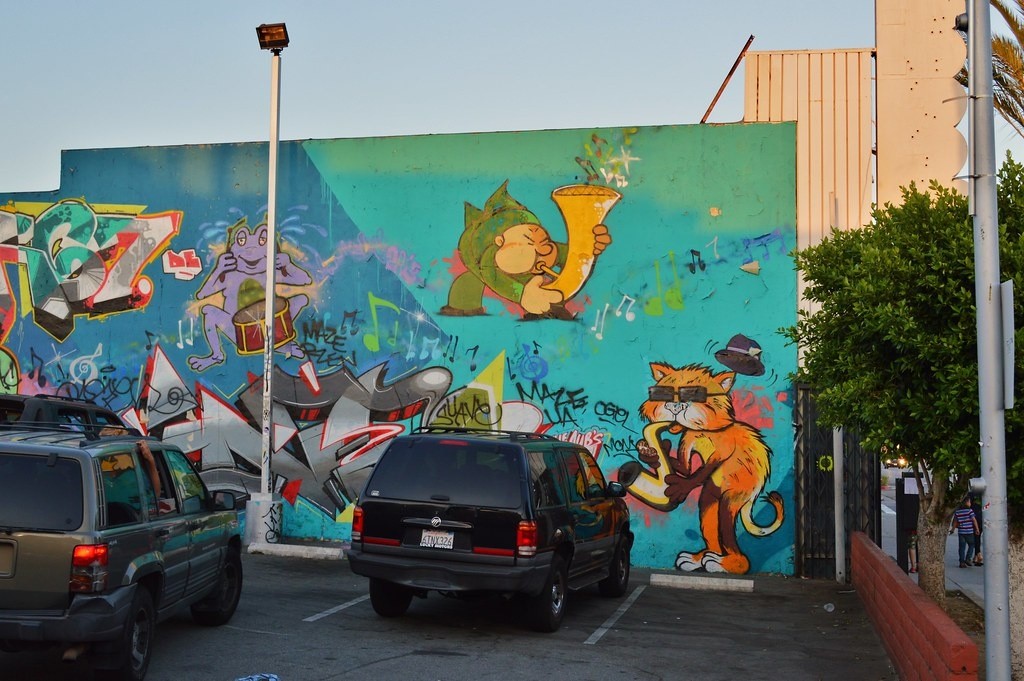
[882,454,906,468]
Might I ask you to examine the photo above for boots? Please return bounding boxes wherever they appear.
[973,553,983,562]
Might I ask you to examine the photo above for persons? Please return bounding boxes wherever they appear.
[907,530,917,573]
[136,440,161,498]
[969,497,983,566]
[948,498,979,567]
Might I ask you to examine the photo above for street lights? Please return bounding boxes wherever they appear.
[255,18,290,544]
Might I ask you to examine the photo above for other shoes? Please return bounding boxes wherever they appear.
[965,560,972,566]
[959,563,966,568]
[908,567,915,573]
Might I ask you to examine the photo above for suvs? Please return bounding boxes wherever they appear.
[0,393,142,440]
[344,426,642,632]
[0,424,246,681]
